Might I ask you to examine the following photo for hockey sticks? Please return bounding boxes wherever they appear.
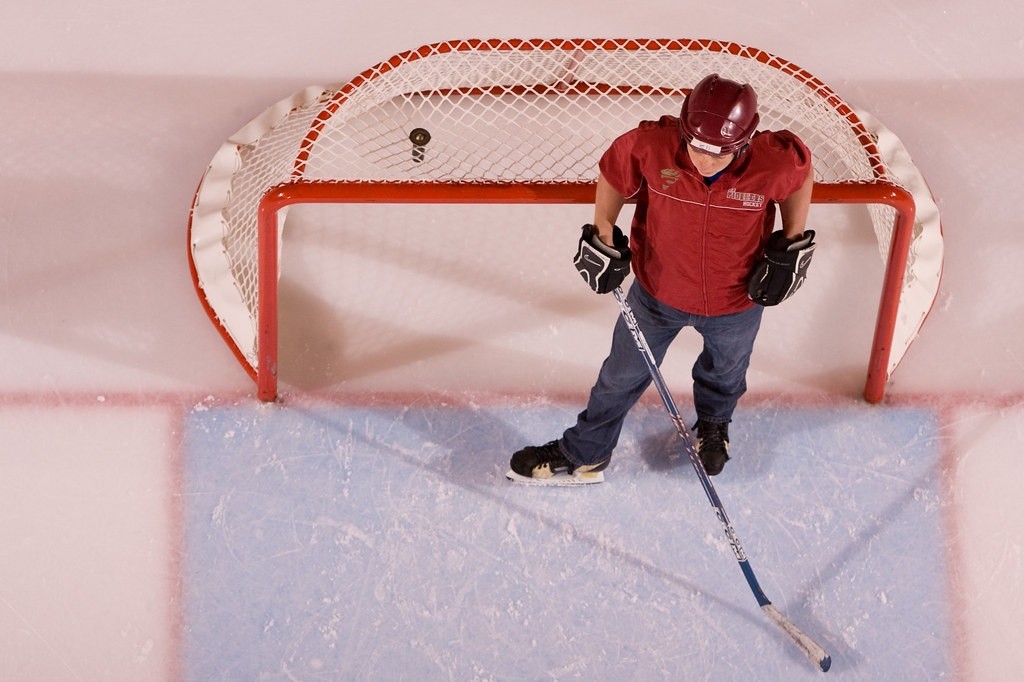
[611,283,832,674]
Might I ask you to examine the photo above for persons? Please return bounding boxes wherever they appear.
[509,71,813,480]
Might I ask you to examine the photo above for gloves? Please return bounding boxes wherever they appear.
[748,228,820,306]
[572,224,631,295]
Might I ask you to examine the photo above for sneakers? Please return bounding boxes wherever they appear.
[505,441,611,487]
[694,421,732,476]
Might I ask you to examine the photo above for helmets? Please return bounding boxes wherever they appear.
[678,72,761,156]
